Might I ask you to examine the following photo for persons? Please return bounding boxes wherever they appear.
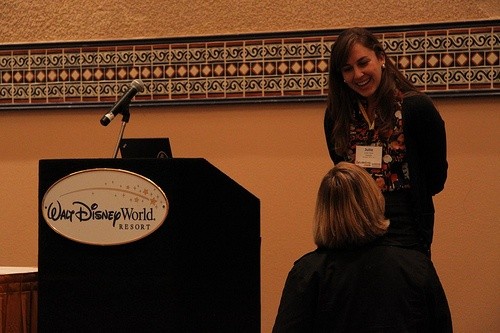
[324,27,448,259]
[272,162,453,333]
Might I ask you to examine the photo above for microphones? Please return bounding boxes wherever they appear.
[100,79,144,126]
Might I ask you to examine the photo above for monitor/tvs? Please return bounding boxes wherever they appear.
[119,137,172,158]
